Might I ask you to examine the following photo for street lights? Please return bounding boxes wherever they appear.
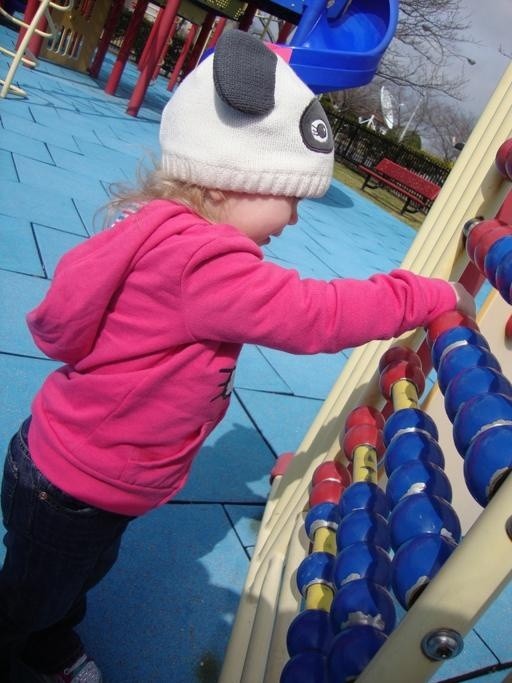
[397,22,478,143]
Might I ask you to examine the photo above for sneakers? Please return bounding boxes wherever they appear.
[53,647,104,682]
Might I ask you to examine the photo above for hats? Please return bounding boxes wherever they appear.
[154,27,338,201]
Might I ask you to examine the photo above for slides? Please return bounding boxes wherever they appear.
[199,0,400,94]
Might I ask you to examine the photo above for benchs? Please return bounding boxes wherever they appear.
[360,155,443,216]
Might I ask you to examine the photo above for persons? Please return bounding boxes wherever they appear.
[1,32,477,682]
